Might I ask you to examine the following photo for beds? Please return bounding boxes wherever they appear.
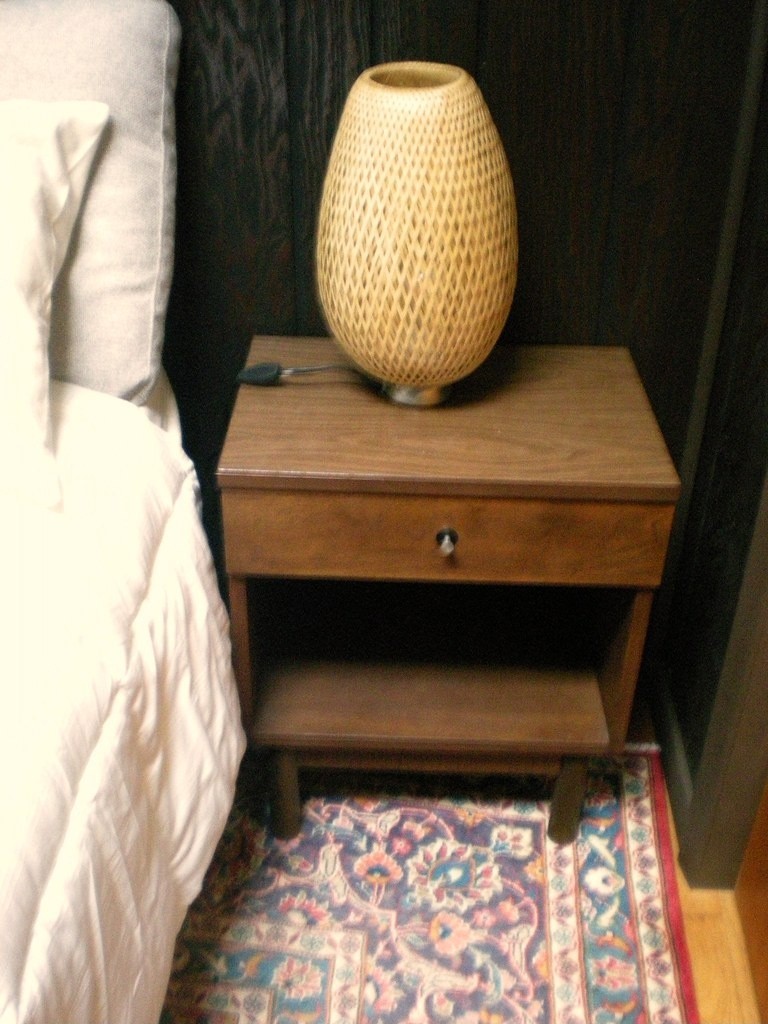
[0,370,252,1024]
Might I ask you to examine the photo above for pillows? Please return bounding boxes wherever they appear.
[0,100,112,460]
[0,0,183,402]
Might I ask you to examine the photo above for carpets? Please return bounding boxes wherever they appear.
[154,745,702,1024]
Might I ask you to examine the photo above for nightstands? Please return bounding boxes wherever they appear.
[213,327,681,845]
